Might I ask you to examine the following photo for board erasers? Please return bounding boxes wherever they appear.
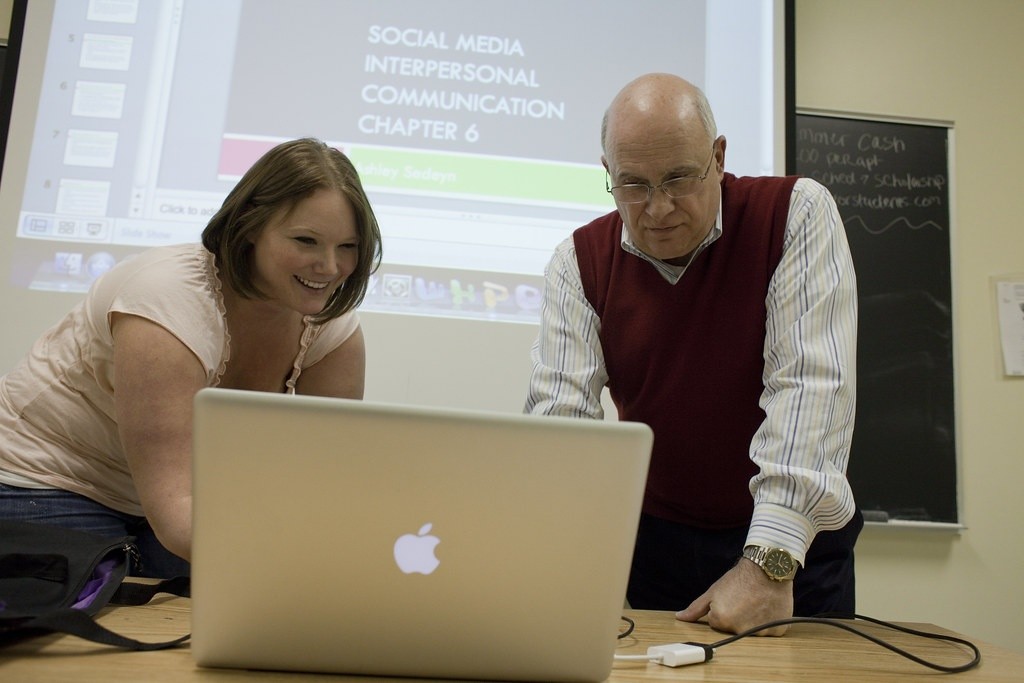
[860,510,889,522]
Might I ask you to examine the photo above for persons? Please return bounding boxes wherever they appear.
[522,74,864,638]
[0,138,384,579]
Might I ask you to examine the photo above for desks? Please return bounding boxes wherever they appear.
[0,576,1024,683]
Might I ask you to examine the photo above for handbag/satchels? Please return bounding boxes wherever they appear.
[0,519,190,654]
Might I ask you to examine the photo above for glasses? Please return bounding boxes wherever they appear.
[605,142,717,203]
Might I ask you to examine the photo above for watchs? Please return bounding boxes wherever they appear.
[741,544,798,582]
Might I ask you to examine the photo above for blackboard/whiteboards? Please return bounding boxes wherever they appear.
[795,105,968,532]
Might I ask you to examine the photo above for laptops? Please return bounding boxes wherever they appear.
[191,389,653,683]
